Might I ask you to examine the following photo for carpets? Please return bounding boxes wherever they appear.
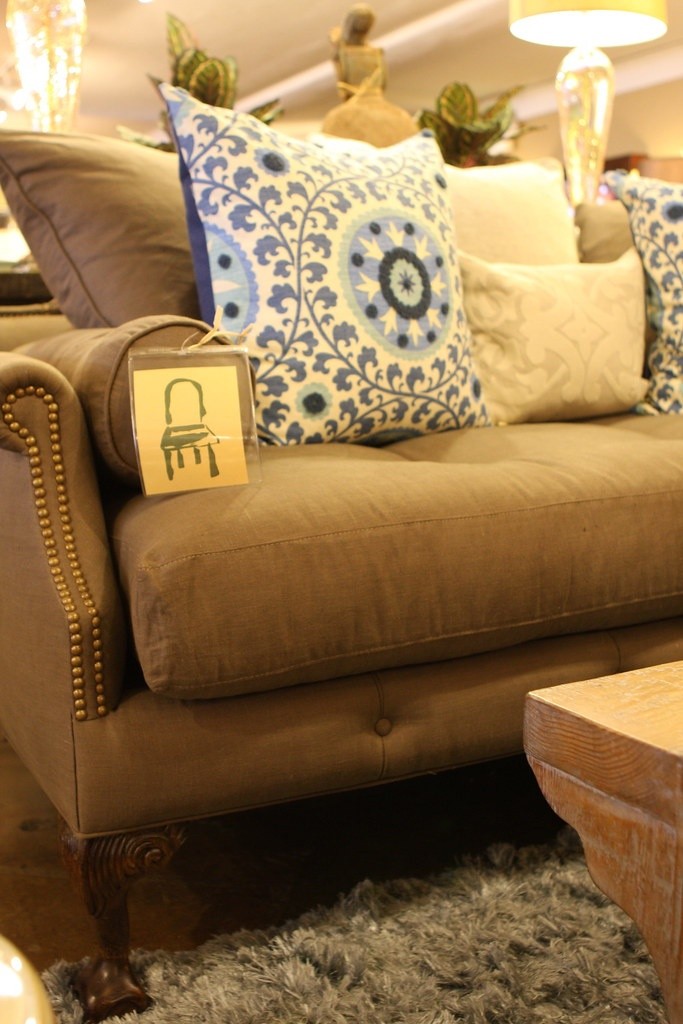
[33,823,672,1024]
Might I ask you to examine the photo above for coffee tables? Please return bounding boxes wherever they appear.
[522,657,683,1024]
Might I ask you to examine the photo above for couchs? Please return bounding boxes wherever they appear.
[0,129,682,1024]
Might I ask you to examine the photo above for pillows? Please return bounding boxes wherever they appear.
[604,168,683,418]
[452,248,653,428]
[157,81,493,448]
[443,155,583,264]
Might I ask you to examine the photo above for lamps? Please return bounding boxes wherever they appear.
[508,0,668,210]
